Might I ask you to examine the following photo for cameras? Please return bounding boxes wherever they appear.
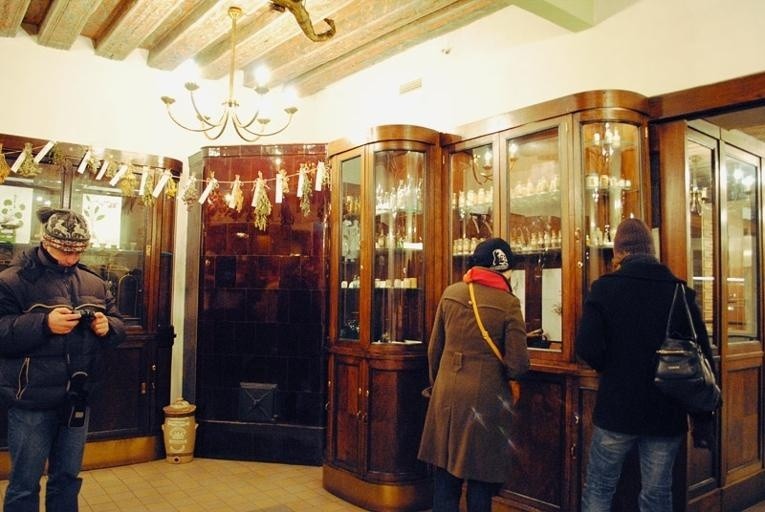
[73,310,96,330]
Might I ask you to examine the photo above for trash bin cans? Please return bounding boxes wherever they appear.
[160,398,199,465]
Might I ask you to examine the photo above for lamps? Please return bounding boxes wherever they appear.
[161,5,299,142]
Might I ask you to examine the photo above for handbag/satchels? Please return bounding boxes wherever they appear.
[647,337,721,412]
[507,379,521,407]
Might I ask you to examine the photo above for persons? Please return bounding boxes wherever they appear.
[414,237,530,511]
[0,205,127,511]
[576,217,725,512]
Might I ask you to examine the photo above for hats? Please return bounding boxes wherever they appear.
[37,206,89,252]
[614,219,655,256]
[473,238,519,273]
